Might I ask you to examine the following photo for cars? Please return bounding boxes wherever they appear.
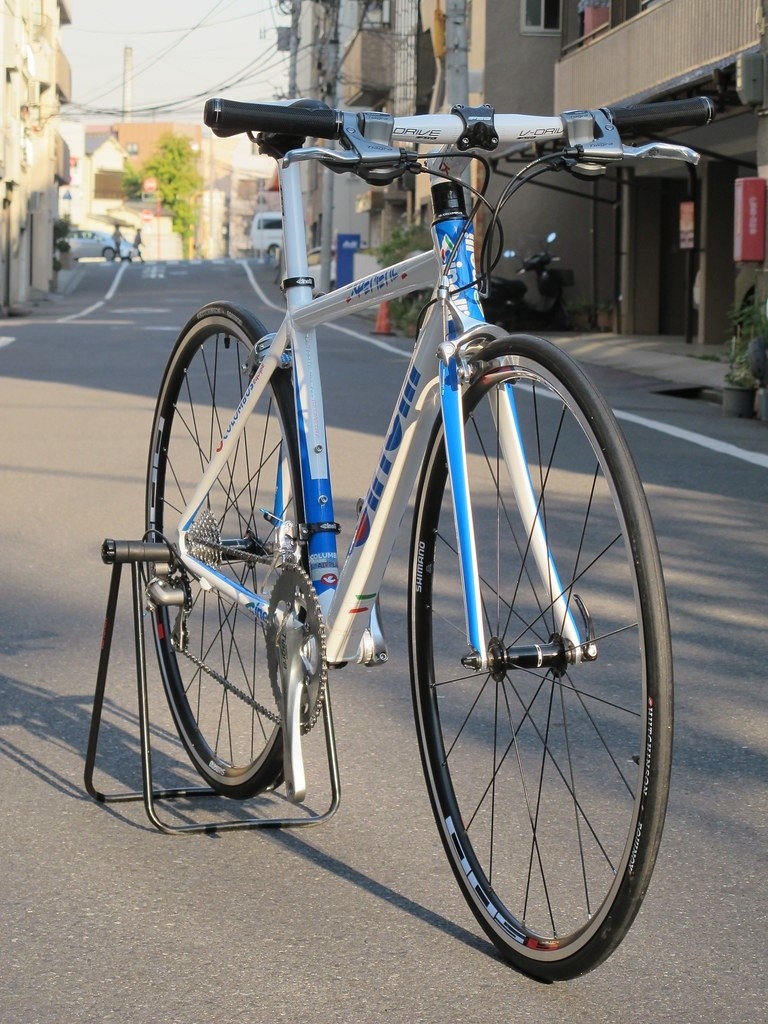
[62,228,139,262]
[280,244,337,303]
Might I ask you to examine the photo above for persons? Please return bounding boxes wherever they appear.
[112,224,128,261]
[127,228,146,261]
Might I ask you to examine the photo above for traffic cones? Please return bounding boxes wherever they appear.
[371,301,398,337]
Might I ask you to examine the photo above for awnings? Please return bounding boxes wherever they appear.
[266,167,279,190]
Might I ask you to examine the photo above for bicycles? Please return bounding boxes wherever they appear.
[85,86,736,981]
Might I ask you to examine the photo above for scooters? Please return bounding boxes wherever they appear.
[479,229,568,329]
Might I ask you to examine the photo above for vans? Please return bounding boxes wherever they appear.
[250,208,285,259]
